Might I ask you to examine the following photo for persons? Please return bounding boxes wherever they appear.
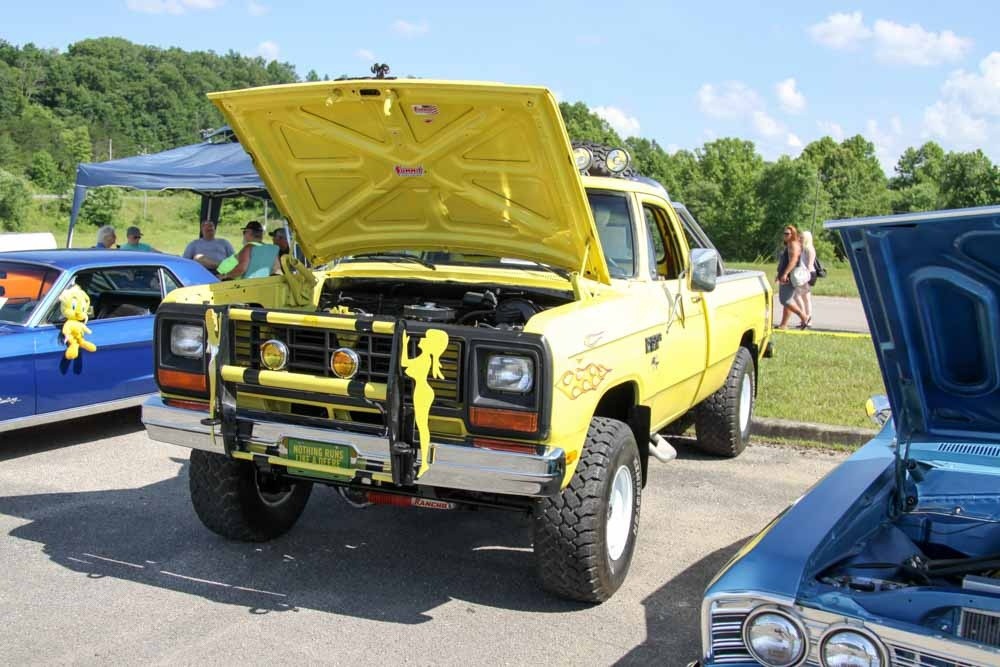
[75,220,305,296]
[774,225,817,329]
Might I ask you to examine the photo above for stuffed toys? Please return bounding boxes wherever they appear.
[59,284,97,359]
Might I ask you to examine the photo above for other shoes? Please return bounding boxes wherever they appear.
[796,325,811,328]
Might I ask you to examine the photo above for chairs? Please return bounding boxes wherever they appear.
[241,245,279,279]
[95,303,150,319]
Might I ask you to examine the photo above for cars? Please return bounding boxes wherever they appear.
[0,249,222,431]
[685,205,1000,666]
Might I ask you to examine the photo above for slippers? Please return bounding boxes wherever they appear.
[802,316,811,330]
[774,325,785,329]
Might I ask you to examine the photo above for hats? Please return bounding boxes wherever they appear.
[269,227,286,236]
[127,226,143,235]
[240,221,263,232]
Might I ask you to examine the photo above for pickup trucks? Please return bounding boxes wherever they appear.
[142,79,774,602]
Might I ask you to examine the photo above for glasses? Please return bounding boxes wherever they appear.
[242,230,254,235]
[129,236,141,239]
[784,232,792,235]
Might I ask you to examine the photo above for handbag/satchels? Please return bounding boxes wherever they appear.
[790,268,811,287]
[814,257,826,278]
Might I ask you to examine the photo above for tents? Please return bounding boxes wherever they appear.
[66,126,297,249]
